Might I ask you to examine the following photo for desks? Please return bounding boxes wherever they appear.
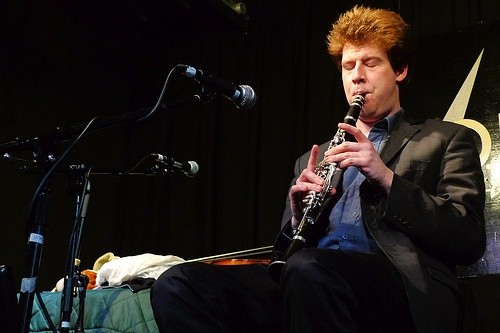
[17,287,159,333]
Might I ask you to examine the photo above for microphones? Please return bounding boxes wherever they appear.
[150,153,199,177]
[177,64,257,111]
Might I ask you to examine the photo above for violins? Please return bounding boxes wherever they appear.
[202,258,271,268]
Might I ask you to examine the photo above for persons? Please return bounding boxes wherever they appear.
[146,4,488,333]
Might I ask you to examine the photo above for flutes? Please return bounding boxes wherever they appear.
[268,92,365,285]
